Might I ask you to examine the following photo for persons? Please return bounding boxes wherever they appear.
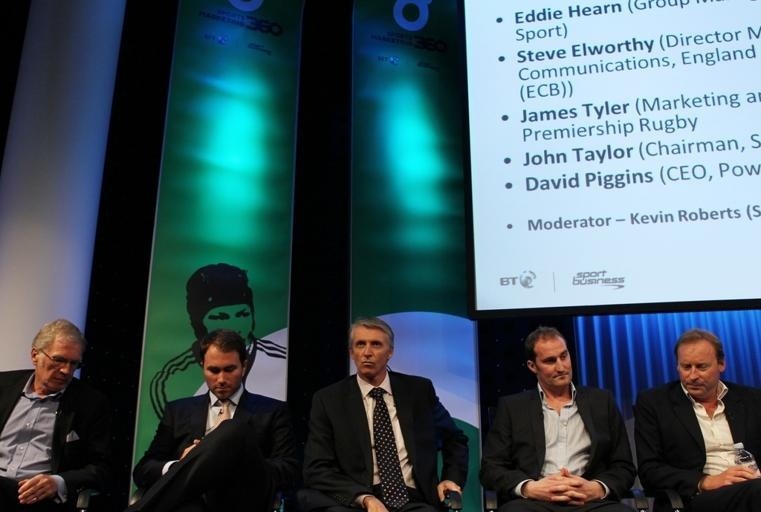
[125,330,304,512]
[300,315,470,511]
[480,327,636,512]
[1,318,107,511]
[635,328,761,511]
[150,262,287,420]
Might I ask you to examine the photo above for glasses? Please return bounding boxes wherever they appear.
[39,348,82,369]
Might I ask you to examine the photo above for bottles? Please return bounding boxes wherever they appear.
[733,441,761,477]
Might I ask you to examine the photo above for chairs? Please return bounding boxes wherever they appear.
[73,412,761,512]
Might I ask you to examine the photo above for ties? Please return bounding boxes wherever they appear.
[218,400,231,430]
[369,387,411,509]
